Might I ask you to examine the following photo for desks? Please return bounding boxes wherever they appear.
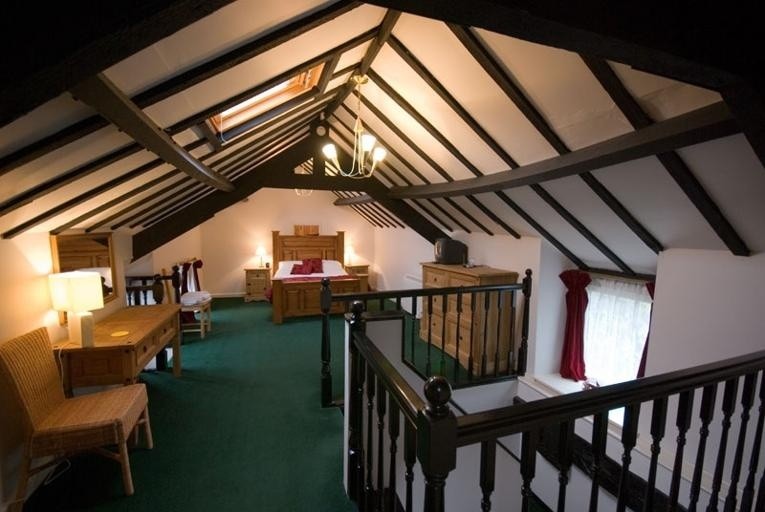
[54,303,186,437]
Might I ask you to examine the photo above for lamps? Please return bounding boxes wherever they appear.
[346,245,355,264]
[254,245,268,268]
[316,67,388,180]
[46,269,107,349]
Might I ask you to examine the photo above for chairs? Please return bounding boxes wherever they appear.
[0,325,157,501]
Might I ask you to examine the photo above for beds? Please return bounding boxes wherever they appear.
[269,223,370,328]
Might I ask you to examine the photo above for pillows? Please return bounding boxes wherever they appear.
[304,258,322,271]
[290,264,311,275]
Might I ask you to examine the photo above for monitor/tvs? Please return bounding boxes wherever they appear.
[434,238,467,264]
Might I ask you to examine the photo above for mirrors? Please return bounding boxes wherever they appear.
[48,231,120,312]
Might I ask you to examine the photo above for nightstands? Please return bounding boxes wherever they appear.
[345,262,371,275]
[244,265,272,304]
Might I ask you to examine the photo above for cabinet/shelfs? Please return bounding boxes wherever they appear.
[417,258,524,378]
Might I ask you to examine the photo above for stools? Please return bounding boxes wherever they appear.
[179,290,213,341]
